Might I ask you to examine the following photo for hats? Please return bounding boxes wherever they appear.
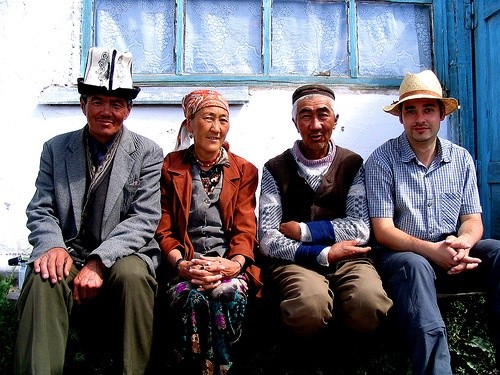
[77,45,141,99]
[383,69,458,116]
[292,85,336,103]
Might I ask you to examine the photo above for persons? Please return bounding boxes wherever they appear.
[156,90,263,375]
[15,47,164,375]
[257,84,393,375]
[364,70,500,375]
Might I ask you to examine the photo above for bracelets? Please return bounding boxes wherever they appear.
[233,259,243,268]
[174,258,185,276]
